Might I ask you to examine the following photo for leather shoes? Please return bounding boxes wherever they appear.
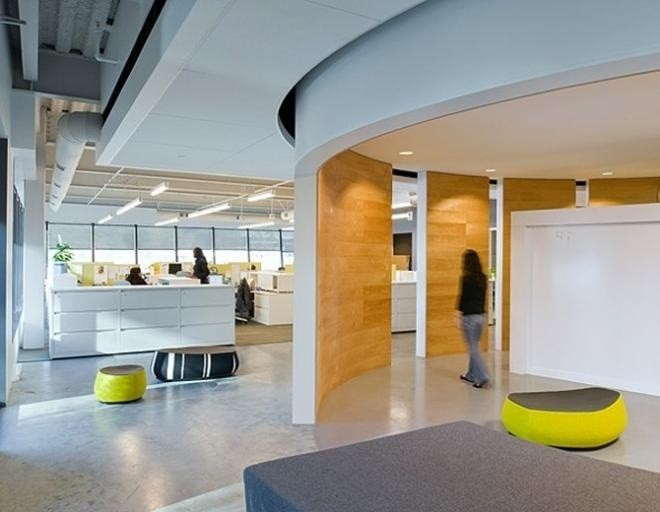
[461,374,474,383]
[472,383,483,388]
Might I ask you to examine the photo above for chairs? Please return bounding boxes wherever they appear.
[113,280,130,286]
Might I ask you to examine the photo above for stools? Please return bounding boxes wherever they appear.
[151,345,239,380]
[95,366,152,403]
[501,386,628,451]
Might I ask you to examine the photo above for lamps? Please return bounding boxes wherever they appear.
[247,188,276,202]
[236,192,276,229]
[96,214,114,224]
[149,181,169,199]
[116,196,144,217]
[186,201,231,219]
[151,213,181,227]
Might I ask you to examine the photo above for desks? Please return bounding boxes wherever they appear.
[243,420,659,511]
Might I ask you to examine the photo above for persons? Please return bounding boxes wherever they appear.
[192,248,210,284]
[454,249,490,389]
[126,268,146,284]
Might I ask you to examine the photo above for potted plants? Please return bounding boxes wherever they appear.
[51,234,75,274]
